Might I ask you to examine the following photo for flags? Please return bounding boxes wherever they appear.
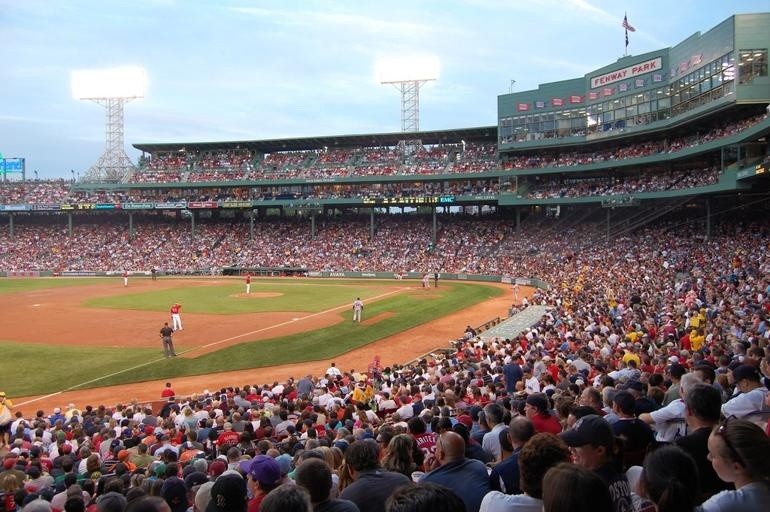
[622,16,636,33]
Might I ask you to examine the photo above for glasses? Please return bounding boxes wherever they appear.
[715,414,749,467]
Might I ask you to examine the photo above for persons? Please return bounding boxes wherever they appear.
[1,109,770,512]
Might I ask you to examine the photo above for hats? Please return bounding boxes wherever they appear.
[95,418,313,511]
[668,356,679,362]
[526,393,548,409]
[725,363,756,386]
[619,379,643,391]
[557,415,612,448]
